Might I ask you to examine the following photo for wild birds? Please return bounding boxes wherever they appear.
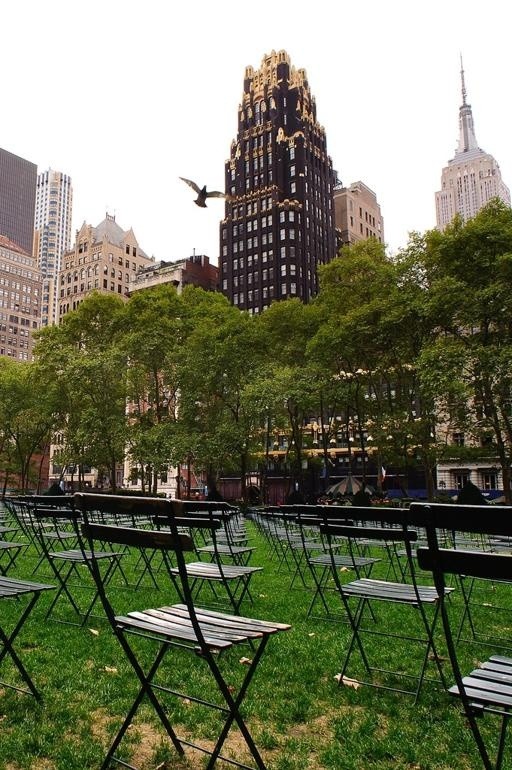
[180,176,237,208]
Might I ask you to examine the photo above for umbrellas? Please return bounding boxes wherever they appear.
[326,476,373,496]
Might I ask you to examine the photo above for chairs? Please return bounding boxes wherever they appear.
[291,504,385,630]
[73,491,296,769]
[408,501,511,769]
[450,501,510,654]
[150,495,265,661]
[2,571,60,707]
[27,494,133,630]
[312,501,456,709]
[2,487,512,603]
